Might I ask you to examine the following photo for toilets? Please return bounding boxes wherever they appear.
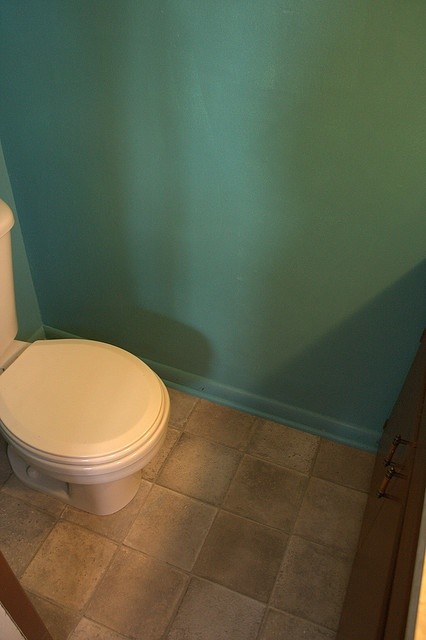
[0,197,171,516]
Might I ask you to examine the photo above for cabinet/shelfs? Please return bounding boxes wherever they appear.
[336,325,425,640]
